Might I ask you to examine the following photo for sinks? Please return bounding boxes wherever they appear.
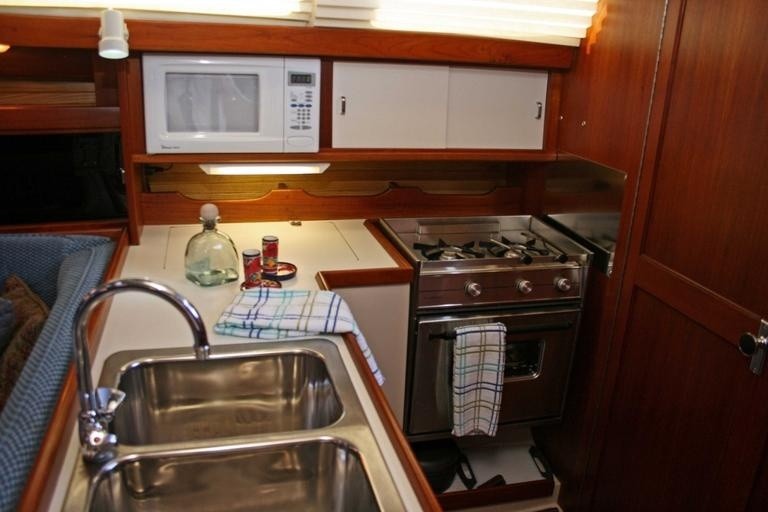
[105,346,344,447]
[80,434,384,512]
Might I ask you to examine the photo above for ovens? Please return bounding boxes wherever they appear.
[406,309,583,434]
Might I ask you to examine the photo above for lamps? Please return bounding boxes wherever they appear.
[92,7,130,61]
[196,163,329,180]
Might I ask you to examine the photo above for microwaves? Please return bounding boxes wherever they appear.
[139,51,320,154]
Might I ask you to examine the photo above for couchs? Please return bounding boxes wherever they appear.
[0,228,125,512]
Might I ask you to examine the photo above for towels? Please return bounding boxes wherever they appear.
[216,283,386,389]
[452,320,506,438]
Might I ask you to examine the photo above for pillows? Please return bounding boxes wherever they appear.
[0,272,48,403]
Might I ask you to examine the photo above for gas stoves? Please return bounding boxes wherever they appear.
[379,215,592,303]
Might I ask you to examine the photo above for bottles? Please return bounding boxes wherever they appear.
[181,203,240,285]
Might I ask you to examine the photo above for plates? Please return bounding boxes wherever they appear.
[241,279,282,293]
[268,262,297,281]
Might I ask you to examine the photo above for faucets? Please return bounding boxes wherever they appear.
[68,275,213,430]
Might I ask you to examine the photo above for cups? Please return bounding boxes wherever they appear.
[242,249,263,286]
[262,235,278,275]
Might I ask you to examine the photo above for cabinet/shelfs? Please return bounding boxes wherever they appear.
[0,13,139,245]
[328,61,558,159]
